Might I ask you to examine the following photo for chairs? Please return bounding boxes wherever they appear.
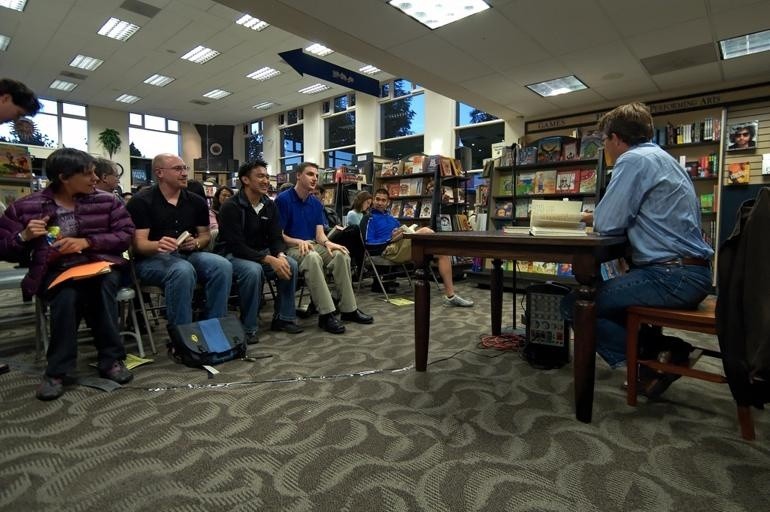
[296,270,331,307]
[229,272,276,302]
[127,242,205,354]
[403,259,441,291]
[27,252,146,360]
[626,187,770,441]
[0,269,49,354]
[357,229,414,303]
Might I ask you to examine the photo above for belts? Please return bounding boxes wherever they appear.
[647,256,711,267]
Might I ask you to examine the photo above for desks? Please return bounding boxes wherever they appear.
[403,230,631,424]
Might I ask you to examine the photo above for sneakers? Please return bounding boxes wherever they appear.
[271,318,304,334]
[443,294,475,307]
[246,330,258,345]
[371,284,397,294]
[35,373,66,401]
[98,360,134,384]
[384,281,400,288]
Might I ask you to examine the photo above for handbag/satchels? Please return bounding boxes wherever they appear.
[167,315,247,368]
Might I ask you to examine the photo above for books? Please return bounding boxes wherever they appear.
[651,118,720,281]
[381,155,623,282]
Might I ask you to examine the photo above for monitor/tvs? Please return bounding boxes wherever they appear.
[594,147,608,207]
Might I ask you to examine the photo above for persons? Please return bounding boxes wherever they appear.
[0,79,40,124]
[94,155,153,332]
[214,160,303,344]
[359,189,472,307]
[560,103,714,397]
[729,126,755,149]
[274,162,375,333]
[1,148,135,399]
[125,153,234,361]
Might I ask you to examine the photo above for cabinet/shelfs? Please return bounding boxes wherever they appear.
[278,178,373,228]
[464,147,603,293]
[603,109,728,287]
[371,153,472,280]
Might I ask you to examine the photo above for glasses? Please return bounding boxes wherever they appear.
[160,164,190,172]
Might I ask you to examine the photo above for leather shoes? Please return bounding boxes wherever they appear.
[622,335,694,400]
[337,309,374,322]
[318,314,346,334]
